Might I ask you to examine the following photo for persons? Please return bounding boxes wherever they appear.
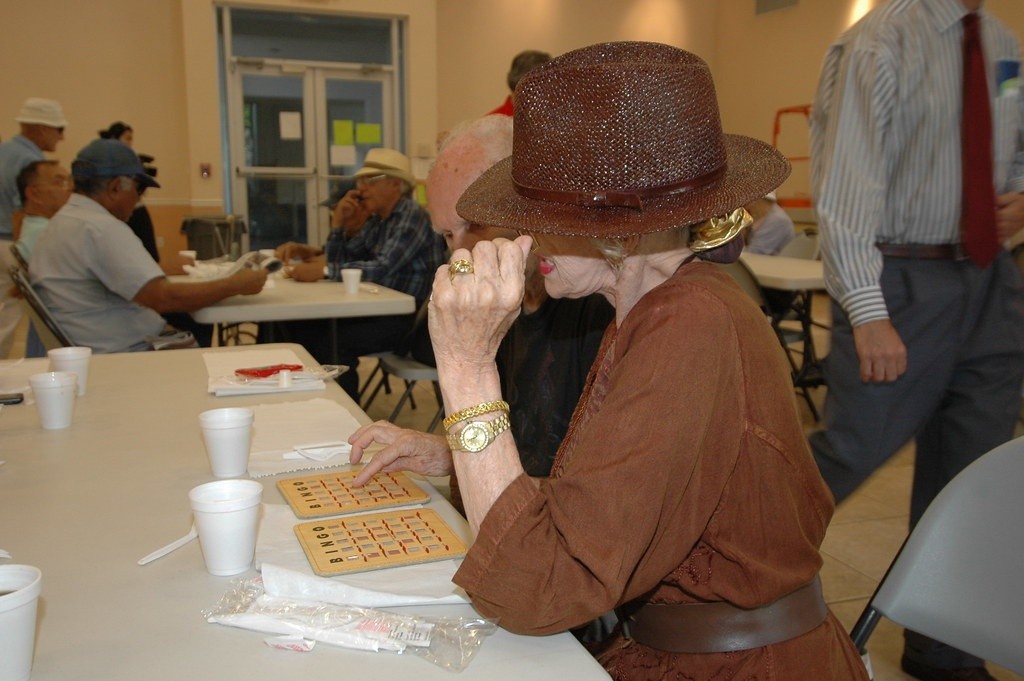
[256,179,369,382]
[289,148,448,405]
[0,98,268,355]
[746,188,800,327]
[425,113,623,654]
[805,0,1024,681]
[488,50,553,117]
[349,41,871,681]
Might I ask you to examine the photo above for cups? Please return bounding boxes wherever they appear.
[0,564,42,679]
[48,346,91,395]
[179,250,197,273]
[198,407,254,477]
[188,479,264,576]
[341,269,362,295]
[28,372,76,429]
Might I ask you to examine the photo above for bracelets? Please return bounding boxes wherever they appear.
[443,400,510,431]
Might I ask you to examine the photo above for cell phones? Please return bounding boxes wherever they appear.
[235,364,302,378]
[0,394,24,405]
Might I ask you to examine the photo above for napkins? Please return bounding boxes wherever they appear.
[201,348,326,396]
[245,398,373,478]
[254,503,472,609]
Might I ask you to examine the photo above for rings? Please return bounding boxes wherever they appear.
[448,258,474,282]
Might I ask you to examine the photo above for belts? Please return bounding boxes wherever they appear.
[874,240,972,261]
[613,571,829,654]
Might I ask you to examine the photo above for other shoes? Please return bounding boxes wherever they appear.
[901,652,999,681]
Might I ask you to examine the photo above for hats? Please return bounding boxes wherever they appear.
[319,180,363,210]
[351,147,416,189]
[456,41,793,240]
[71,140,161,190]
[14,97,68,128]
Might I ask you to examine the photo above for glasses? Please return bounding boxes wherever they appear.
[58,128,64,133]
[126,174,147,194]
[356,174,394,189]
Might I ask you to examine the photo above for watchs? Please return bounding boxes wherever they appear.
[446,411,511,454]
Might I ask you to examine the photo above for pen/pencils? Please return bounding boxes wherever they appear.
[243,260,256,268]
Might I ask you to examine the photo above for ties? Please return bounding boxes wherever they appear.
[960,13,1001,268]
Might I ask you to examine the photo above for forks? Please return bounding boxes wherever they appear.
[296,448,351,461]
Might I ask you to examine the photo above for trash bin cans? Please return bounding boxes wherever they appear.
[180,216,246,266]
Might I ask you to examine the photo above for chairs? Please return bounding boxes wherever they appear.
[774,226,822,392]
[358,290,445,432]
[851,437,1024,681]
[9,244,28,270]
[9,264,76,347]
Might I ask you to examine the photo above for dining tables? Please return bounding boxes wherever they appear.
[739,253,826,426]
[0,341,619,681]
[164,259,416,388]
